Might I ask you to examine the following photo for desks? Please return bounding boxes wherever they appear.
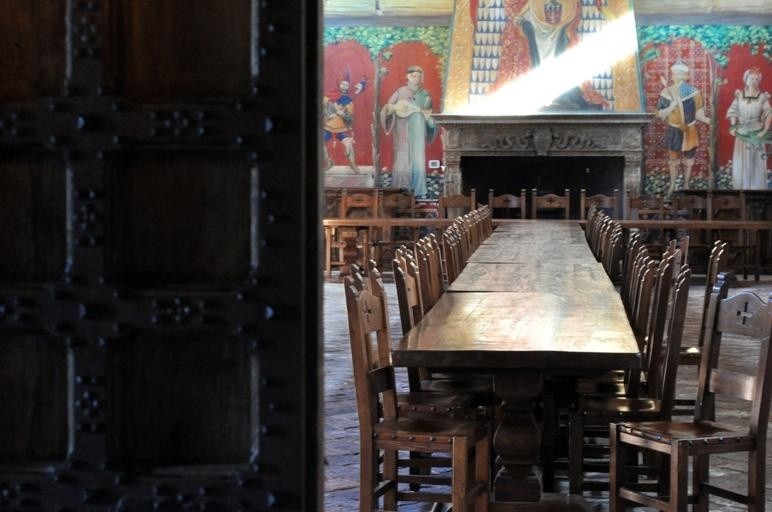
[389,292,640,512]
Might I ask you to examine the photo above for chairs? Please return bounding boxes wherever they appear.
[568,264,692,494]
[350,260,504,496]
[392,205,493,375]
[609,269,770,512]
[323,187,772,282]
[643,240,728,421]
[582,202,658,352]
[343,276,494,512]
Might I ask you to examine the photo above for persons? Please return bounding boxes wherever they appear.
[655,59,711,197]
[323,65,363,175]
[726,66,771,192]
[380,65,438,205]
